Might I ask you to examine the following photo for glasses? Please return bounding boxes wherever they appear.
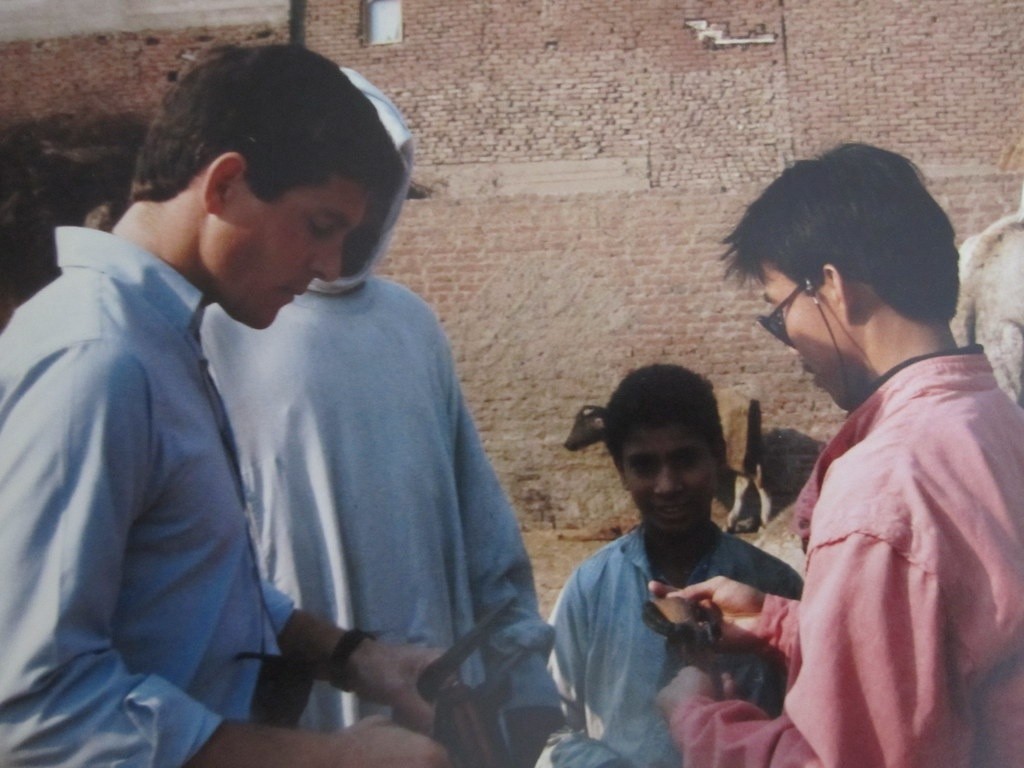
[753,283,815,346]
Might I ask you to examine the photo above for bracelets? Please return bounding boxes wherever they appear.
[331,627,375,690]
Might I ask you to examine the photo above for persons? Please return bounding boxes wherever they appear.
[0,37,458,768]
[547,364,803,768]
[646,143,1024,768]
[199,68,563,767]
